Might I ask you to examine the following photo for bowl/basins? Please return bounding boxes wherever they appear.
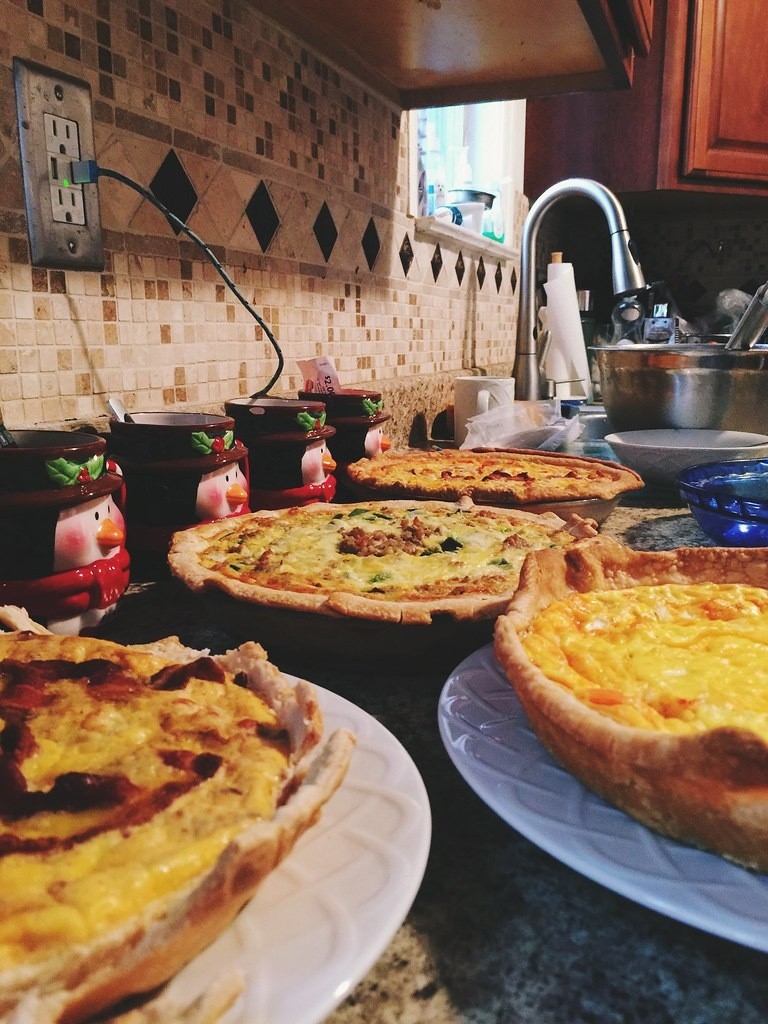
[603,425,768,490]
[674,457,768,548]
[446,188,497,210]
[585,342,768,434]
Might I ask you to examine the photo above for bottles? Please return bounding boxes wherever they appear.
[430,204,469,226]
[426,183,436,216]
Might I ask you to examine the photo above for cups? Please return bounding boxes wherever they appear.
[296,386,392,462]
[454,201,485,234]
[222,398,336,509]
[0,431,137,639]
[110,413,252,575]
[454,376,516,444]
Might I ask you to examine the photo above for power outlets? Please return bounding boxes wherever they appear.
[14,58,106,269]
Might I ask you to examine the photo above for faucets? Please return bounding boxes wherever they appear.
[513,175,649,399]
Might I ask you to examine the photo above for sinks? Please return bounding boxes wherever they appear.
[570,410,618,447]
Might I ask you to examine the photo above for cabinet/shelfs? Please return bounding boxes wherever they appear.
[524,0,768,200]
[254,0,654,111]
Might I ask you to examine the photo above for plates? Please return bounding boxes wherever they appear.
[438,641,768,954]
[113,673,431,1024]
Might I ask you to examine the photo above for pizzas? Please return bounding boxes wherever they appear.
[343,446,645,503]
[494,532,768,876]
[0,601,355,1024]
[168,497,598,627]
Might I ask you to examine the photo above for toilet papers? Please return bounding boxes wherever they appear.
[538,261,592,401]
